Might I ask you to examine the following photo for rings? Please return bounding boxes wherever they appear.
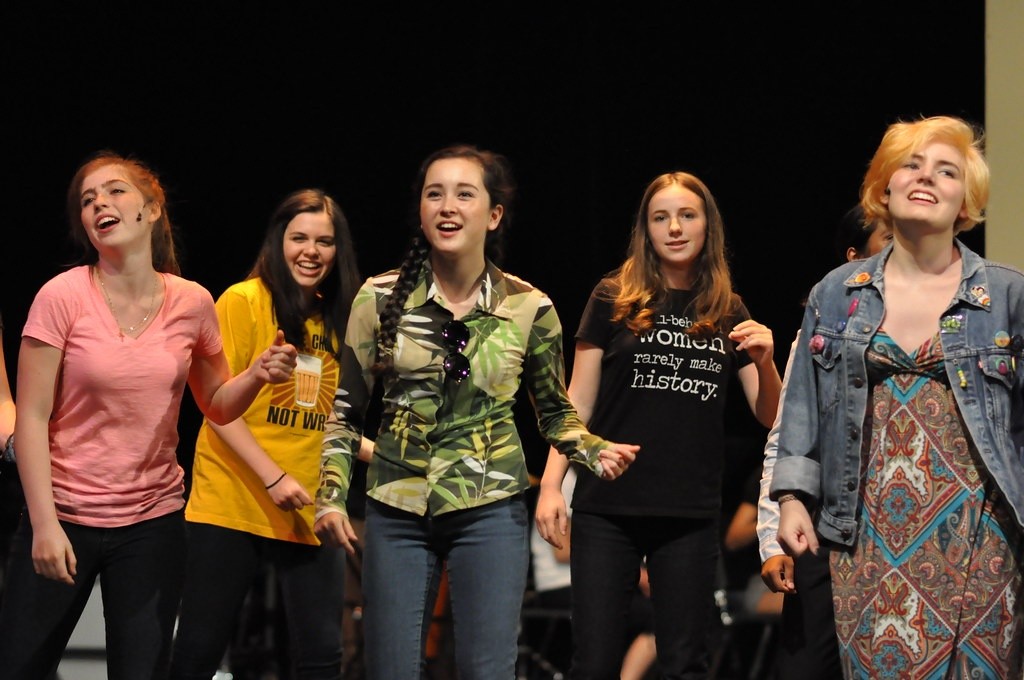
[618,459,625,467]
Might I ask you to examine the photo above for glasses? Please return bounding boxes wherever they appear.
[441,320,469,383]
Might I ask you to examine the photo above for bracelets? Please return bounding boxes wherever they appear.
[265,472,286,489]
[4,434,14,447]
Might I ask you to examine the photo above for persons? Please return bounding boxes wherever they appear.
[0,113,1024,680]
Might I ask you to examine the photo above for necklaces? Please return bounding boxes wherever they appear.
[97,263,157,333]
